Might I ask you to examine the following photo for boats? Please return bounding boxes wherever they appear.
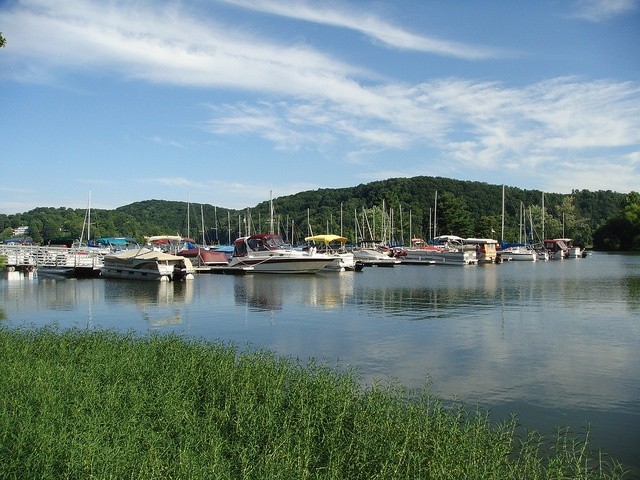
[226,234,343,274]
[463,237,571,263]
[348,249,402,268]
